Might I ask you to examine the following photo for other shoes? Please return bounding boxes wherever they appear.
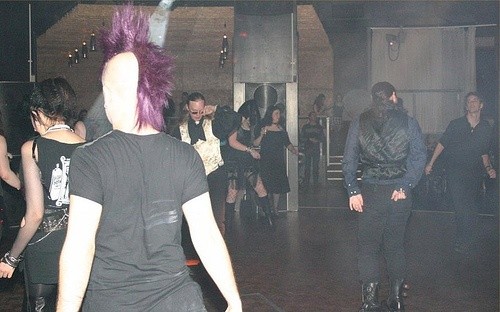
[273,212,281,219]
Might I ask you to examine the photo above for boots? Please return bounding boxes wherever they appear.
[224,202,235,245]
[357,280,384,312]
[384,279,407,312]
[260,194,276,232]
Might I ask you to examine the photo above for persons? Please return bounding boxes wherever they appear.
[163,92,299,239]
[299,93,351,192]
[343,82,427,311]
[56,1,242,312]
[0,77,97,312]
[425,92,500,253]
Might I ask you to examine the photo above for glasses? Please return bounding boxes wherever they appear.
[188,107,204,114]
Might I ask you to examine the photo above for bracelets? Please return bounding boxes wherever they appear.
[290,149,296,151]
[245,146,254,152]
[214,104,217,112]
[3,251,18,268]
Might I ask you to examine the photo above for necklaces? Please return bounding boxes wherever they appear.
[273,123,281,128]
[44,124,72,131]
